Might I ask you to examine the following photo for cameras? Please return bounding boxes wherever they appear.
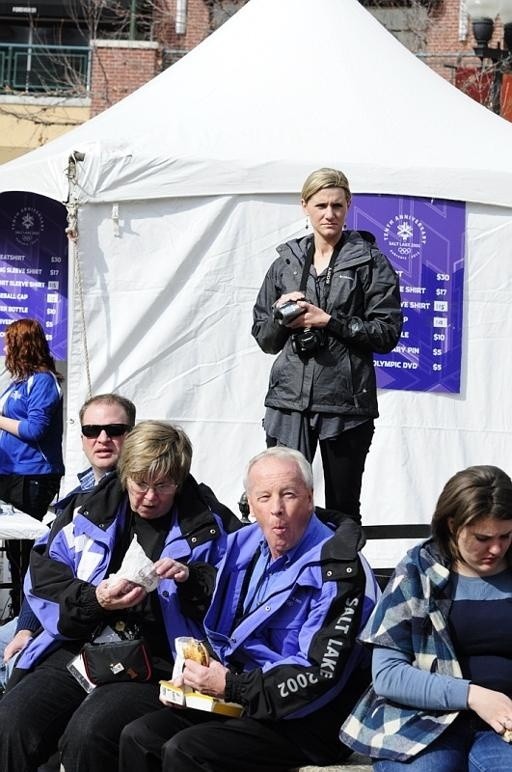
[272,298,313,326]
[291,330,327,354]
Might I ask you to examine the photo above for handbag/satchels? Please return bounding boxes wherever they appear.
[82,638,152,684]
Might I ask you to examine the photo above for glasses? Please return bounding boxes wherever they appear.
[81,423,132,438]
[131,480,178,495]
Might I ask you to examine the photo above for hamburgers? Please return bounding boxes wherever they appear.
[177,636,211,669]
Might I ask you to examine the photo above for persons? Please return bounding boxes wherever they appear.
[250,167,405,528]
[117,446,384,772]
[0,419,228,772]
[338,464,512,771]
[0,318,64,617]
[0,392,137,695]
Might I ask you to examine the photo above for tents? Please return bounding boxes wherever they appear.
[0,0,512,594]
